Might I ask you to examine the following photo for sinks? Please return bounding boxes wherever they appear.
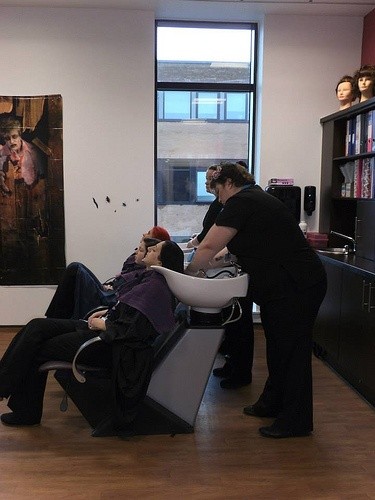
[176,243,194,252]
[182,247,238,266]
[316,247,353,255]
[149,262,249,314]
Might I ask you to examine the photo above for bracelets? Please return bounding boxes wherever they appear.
[190,240,196,246]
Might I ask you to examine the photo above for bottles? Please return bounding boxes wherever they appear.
[298,221,307,239]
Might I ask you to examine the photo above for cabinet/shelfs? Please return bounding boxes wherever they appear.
[319,97,374,263]
[314,249,375,405]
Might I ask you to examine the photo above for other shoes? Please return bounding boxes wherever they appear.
[220,375,253,389]
[1,411,42,426]
[213,366,233,377]
[259,420,312,438]
[243,402,278,417]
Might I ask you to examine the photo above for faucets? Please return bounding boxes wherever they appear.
[330,230,356,252]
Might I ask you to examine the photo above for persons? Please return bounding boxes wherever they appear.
[187,163,327,439]
[187,165,256,392]
[334,64,375,111]
[0,241,185,426]
[44,227,170,320]
